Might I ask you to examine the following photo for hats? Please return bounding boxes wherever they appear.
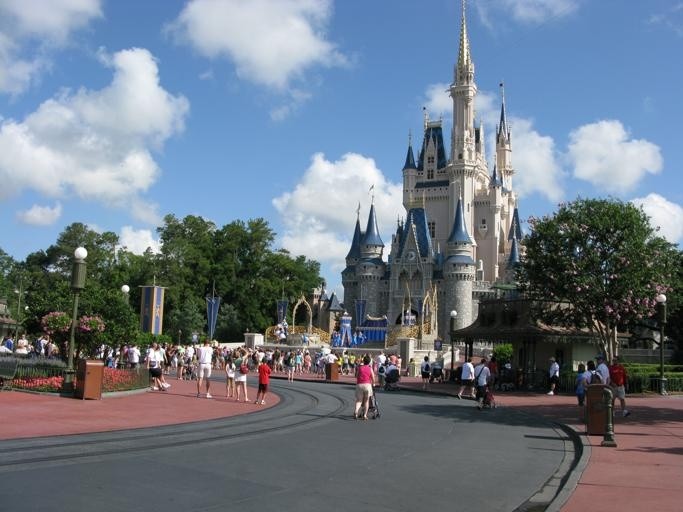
[594,355,604,359]
[549,357,554,361]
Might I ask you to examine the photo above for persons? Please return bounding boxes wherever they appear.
[420,356,430,391]
[226,351,271,403]
[354,355,375,420]
[144,339,219,397]
[575,355,630,418]
[103,343,141,368]
[315,350,402,392]
[548,357,559,396]
[6,334,54,355]
[457,356,496,410]
[219,346,311,382]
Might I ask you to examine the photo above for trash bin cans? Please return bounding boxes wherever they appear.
[326,363,338,381]
[586,384,612,435]
[79,359,103,400]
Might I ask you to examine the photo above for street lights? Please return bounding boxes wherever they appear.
[59,247,89,395]
[122,285,131,294]
[656,294,668,395]
[14,289,29,345]
[450,309,458,383]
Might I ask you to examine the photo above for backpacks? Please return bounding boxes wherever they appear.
[589,370,601,384]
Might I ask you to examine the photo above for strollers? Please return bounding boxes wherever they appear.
[428,367,445,384]
[183,364,198,380]
[484,387,498,409]
[361,389,382,420]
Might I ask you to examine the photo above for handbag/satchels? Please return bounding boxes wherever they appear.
[471,377,477,388]
[550,374,557,384]
[239,364,248,374]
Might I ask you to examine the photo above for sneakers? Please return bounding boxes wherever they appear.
[456,393,462,400]
[622,410,629,417]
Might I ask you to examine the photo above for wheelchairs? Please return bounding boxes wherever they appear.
[383,370,401,391]
